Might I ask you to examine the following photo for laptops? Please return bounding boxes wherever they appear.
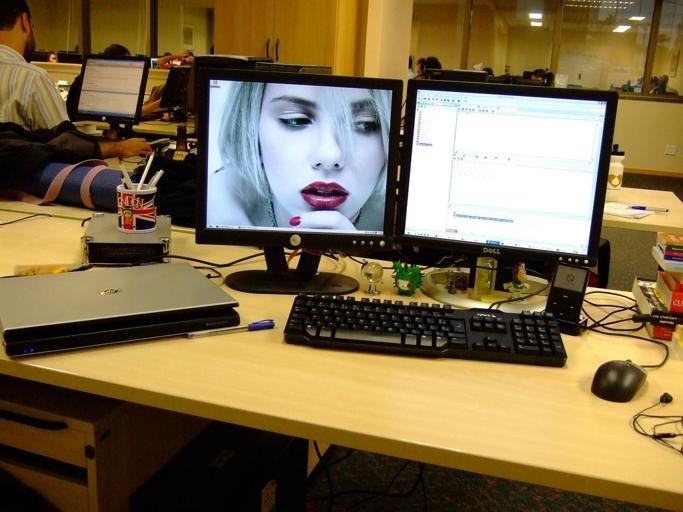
[0,260,240,360]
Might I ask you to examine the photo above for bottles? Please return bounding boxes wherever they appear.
[607,151,625,190]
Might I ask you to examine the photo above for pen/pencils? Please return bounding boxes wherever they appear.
[119,150,164,191]
[185,319,275,339]
[630,206,669,212]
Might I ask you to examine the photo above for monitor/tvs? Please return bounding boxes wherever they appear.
[425,67,489,82]
[75,54,151,135]
[194,64,403,296]
[510,75,543,84]
[394,78,620,314]
[159,63,191,115]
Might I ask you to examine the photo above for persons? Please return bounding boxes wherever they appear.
[220,82,390,231]
[66,43,196,124]
[482,67,494,75]
[46,48,57,62]
[651,75,678,94]
[531,68,555,87]
[406,53,441,79]
[0,2,152,161]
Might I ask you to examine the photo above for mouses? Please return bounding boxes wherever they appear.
[590,359,647,403]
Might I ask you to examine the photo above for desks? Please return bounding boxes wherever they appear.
[0,109,683,512]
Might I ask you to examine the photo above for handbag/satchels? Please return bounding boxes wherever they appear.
[1,120,102,189]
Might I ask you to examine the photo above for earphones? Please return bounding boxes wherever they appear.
[659,392,673,403]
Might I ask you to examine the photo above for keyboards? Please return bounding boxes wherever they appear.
[283,291,568,368]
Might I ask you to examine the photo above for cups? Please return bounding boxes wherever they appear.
[115,184,157,233]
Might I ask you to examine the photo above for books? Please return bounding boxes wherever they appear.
[632,232,683,359]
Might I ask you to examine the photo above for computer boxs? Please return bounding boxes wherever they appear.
[192,54,275,137]
[256,61,333,74]
